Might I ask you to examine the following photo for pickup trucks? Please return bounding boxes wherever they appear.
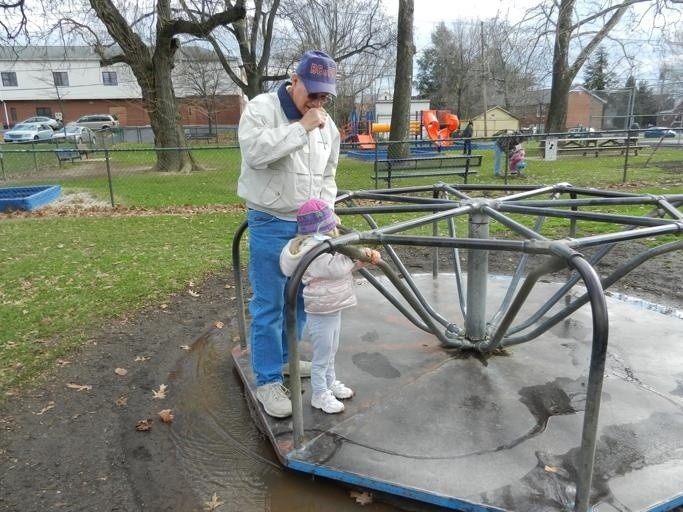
[182,126,216,140]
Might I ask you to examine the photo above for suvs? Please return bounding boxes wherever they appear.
[65,114,119,132]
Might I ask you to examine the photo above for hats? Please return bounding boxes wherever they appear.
[298,199,339,235]
[296,51,338,98]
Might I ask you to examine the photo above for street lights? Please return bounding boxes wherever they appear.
[538,102,545,134]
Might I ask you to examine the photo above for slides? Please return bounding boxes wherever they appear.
[357,134,376,150]
[423,111,460,147]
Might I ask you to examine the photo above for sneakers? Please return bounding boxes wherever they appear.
[281,360,313,378]
[256,382,293,418]
[311,390,345,413]
[328,381,355,399]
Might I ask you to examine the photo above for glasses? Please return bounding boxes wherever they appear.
[303,93,330,103]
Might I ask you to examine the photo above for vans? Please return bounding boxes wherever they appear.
[521,128,530,135]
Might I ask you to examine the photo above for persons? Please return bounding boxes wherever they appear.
[233,47,339,420]
[278,198,382,414]
[493,130,527,178]
[621,122,639,157]
[582,127,601,158]
[460,120,474,156]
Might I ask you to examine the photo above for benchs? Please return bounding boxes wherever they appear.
[536,136,645,159]
[55,149,82,167]
[369,153,483,190]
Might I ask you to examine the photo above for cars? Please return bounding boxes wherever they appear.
[644,127,676,138]
[14,117,57,130]
[565,127,602,141]
[492,128,523,143]
[50,126,96,145]
[3,123,53,144]
